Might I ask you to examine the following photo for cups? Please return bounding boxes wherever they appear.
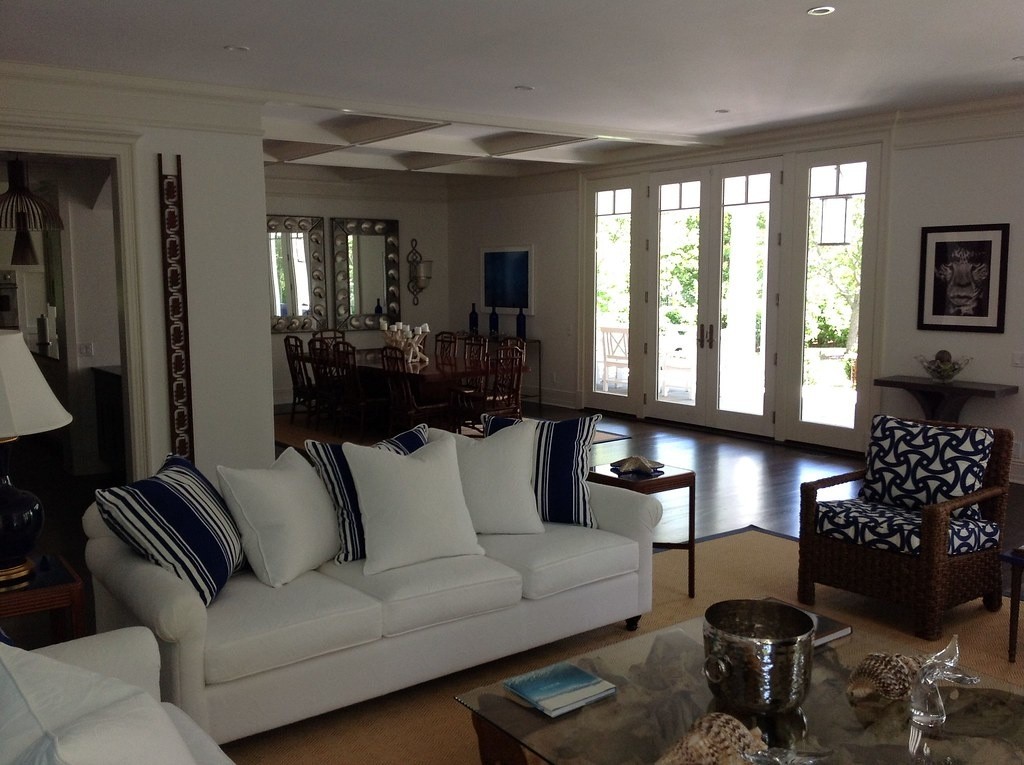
[281,304,288,316]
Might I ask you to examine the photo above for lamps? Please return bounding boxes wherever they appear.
[0,329,74,593]
[405,238,434,306]
[0,149,65,265]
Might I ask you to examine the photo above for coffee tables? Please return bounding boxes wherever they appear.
[455,596,1024,765]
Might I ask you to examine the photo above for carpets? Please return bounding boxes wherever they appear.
[454,415,633,444]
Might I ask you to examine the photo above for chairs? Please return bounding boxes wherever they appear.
[600,326,696,401]
[434,331,458,361]
[465,334,527,429]
[797,414,1014,643]
[284,330,379,442]
[379,345,449,441]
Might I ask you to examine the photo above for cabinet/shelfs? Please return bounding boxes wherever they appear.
[442,334,542,409]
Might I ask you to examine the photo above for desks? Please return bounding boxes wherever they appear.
[0,551,89,651]
[874,375,1018,426]
[18,333,69,366]
[91,363,127,480]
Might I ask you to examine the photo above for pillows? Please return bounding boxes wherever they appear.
[95,451,250,610]
[428,419,543,536]
[214,445,341,588]
[305,423,430,565]
[480,413,603,531]
[0,642,198,765]
[339,431,487,575]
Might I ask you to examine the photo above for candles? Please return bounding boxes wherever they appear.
[380,321,431,339]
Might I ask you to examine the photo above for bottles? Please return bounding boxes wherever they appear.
[489,306,499,338]
[469,303,478,335]
[516,307,526,341]
[375,298,382,314]
[37,314,50,343]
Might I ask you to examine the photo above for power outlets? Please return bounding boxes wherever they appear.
[76,342,94,357]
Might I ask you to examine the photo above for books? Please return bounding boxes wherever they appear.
[762,597,852,648]
[505,659,616,717]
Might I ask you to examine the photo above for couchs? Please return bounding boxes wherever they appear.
[0,624,241,765]
[82,428,667,748]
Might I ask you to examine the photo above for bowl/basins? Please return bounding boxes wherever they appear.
[915,352,975,382]
[704,600,815,715]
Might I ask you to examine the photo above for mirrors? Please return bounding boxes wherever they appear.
[265,214,329,334]
[330,217,402,332]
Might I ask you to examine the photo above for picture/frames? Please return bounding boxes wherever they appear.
[917,223,1011,334]
[479,244,535,318]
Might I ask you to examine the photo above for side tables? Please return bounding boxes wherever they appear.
[585,456,696,599]
